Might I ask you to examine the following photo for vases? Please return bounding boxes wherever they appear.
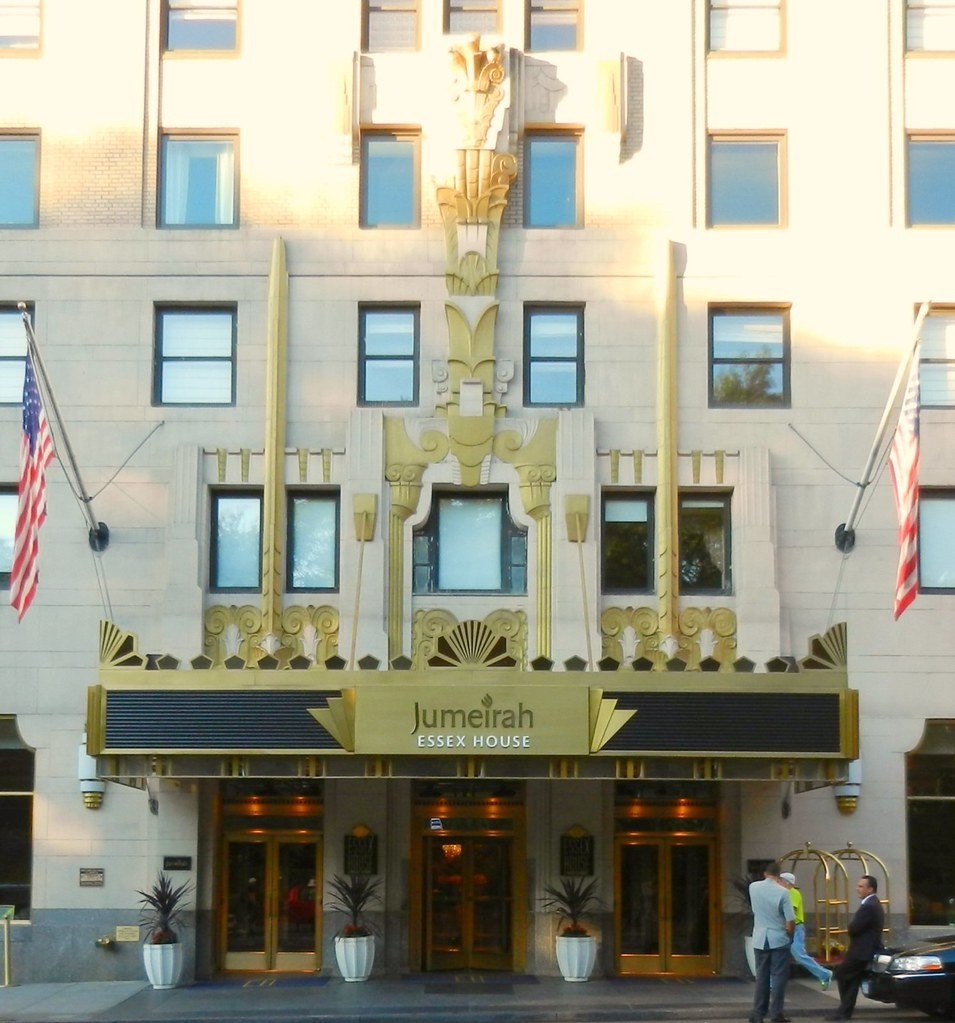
[335,934,375,983]
[142,942,183,989]
[555,934,598,983]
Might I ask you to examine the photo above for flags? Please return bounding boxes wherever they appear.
[888,343,921,621]
[9,354,55,624]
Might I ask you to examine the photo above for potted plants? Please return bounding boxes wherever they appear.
[728,868,765,981]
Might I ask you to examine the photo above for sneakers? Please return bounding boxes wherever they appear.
[821,970,833,991]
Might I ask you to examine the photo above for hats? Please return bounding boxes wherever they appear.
[780,873,796,885]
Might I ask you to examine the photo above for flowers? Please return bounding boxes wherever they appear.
[528,872,611,936]
[135,871,197,943]
[323,871,384,937]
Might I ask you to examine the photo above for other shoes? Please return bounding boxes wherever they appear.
[825,1013,850,1020]
[772,1018,791,1023]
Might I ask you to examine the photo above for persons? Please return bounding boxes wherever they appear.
[825,876,883,1021]
[778,873,832,992]
[749,863,796,1023]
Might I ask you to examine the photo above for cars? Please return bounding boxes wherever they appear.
[865,936,954,1016]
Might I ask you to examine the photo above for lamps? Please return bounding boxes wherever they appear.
[144,757,158,815]
[834,754,864,815]
[79,733,105,808]
[442,844,462,858]
[780,769,794,826]
